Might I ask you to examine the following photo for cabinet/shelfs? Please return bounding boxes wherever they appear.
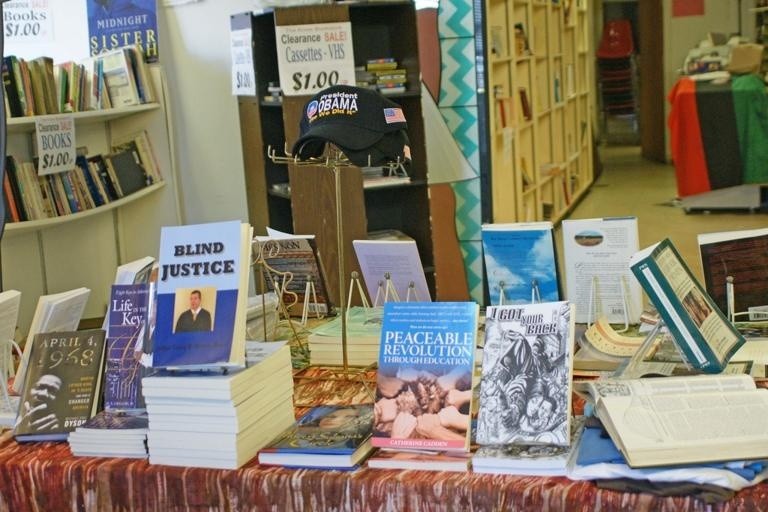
[248,6,298,237]
[346,0,438,301]
[0,98,176,234]
[480,0,602,234]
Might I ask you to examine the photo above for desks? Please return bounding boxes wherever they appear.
[0,305,768,512]
[669,67,766,216]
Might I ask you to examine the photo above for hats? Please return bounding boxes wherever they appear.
[291,85,409,168]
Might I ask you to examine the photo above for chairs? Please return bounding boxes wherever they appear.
[592,17,643,151]
